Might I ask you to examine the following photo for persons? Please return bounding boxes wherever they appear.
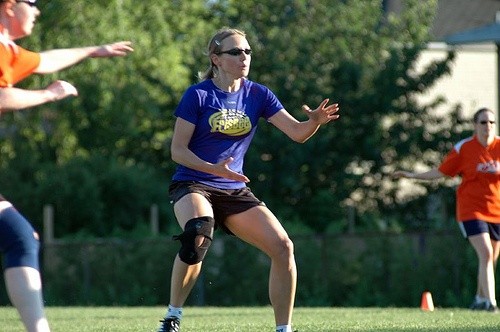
[157,26,341,332]
[0,0,134,332]
[392,106,500,312]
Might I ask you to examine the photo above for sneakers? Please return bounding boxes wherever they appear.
[158,317,182,332]
[485,300,496,311]
[470,297,485,310]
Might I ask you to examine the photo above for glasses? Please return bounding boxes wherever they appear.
[216,49,252,56]
[15,0,37,7]
[480,120,495,124]
